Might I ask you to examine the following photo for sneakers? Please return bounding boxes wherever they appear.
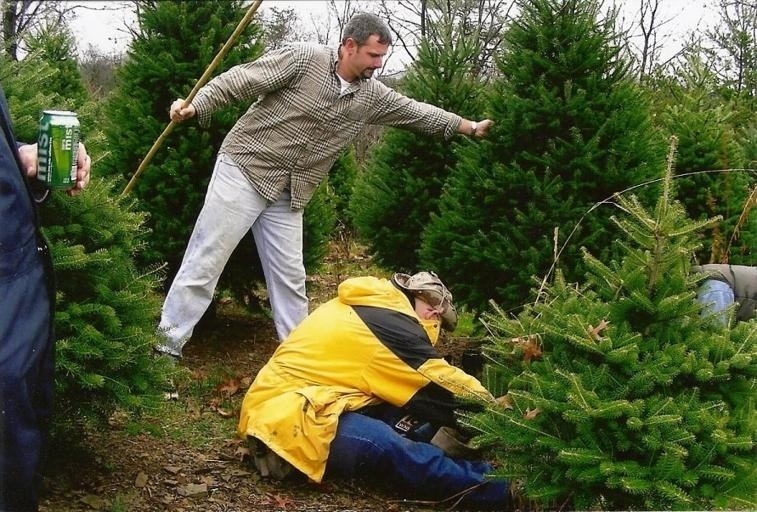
[508,474,608,512]
[152,349,181,401]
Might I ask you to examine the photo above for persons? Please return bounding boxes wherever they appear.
[690,263,757,334]
[151,13,498,400]
[239,269,556,512]
[0,84,91,512]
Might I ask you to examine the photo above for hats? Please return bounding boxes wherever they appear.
[392,269,459,332]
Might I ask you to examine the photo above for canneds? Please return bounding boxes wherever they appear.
[35,110,81,190]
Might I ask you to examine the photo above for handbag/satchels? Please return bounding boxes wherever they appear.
[246,433,295,489]
